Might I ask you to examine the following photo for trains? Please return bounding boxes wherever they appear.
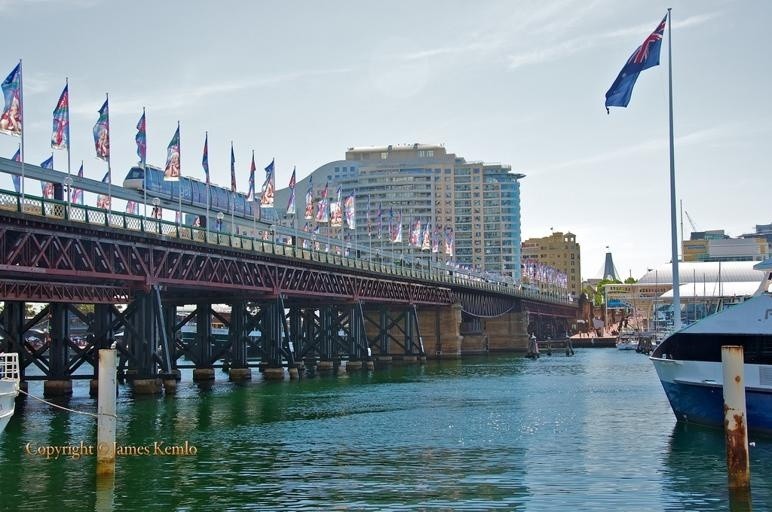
[122,163,282,225]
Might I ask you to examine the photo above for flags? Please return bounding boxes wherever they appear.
[127,112,456,263]
[0,62,23,137]
[10,149,21,196]
[93,101,110,163]
[72,166,83,205]
[96,170,111,212]
[521,255,568,288]
[50,86,67,152]
[603,14,669,113]
[39,156,54,201]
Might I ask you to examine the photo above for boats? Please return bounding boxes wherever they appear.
[646,252,772,436]
[0,352,20,435]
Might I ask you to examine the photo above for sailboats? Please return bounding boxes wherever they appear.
[616,266,673,357]
